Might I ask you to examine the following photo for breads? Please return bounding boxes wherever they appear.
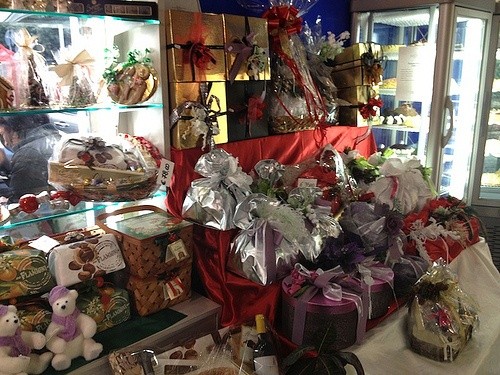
[105,62,148,105]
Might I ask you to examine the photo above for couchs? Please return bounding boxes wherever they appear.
[165,126,479,356]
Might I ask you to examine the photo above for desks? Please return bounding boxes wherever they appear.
[40,294,221,375]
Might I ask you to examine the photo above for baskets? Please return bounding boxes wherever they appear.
[407,277,474,360]
[270,112,322,134]
[95,204,193,279]
[126,260,194,317]
[59,133,162,202]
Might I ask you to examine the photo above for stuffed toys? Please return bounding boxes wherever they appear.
[0,304,53,375]
[45,286,102,370]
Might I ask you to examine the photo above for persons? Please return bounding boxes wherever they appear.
[0,113,86,239]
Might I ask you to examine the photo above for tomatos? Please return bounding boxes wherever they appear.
[0,251,40,330]
[81,276,114,330]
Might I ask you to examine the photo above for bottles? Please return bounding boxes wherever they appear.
[253,314,280,375]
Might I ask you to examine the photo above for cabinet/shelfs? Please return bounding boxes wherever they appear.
[0,9,170,232]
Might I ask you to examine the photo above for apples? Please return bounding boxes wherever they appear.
[19,194,39,214]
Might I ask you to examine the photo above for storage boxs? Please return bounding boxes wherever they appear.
[166,9,271,150]
[329,43,383,127]
